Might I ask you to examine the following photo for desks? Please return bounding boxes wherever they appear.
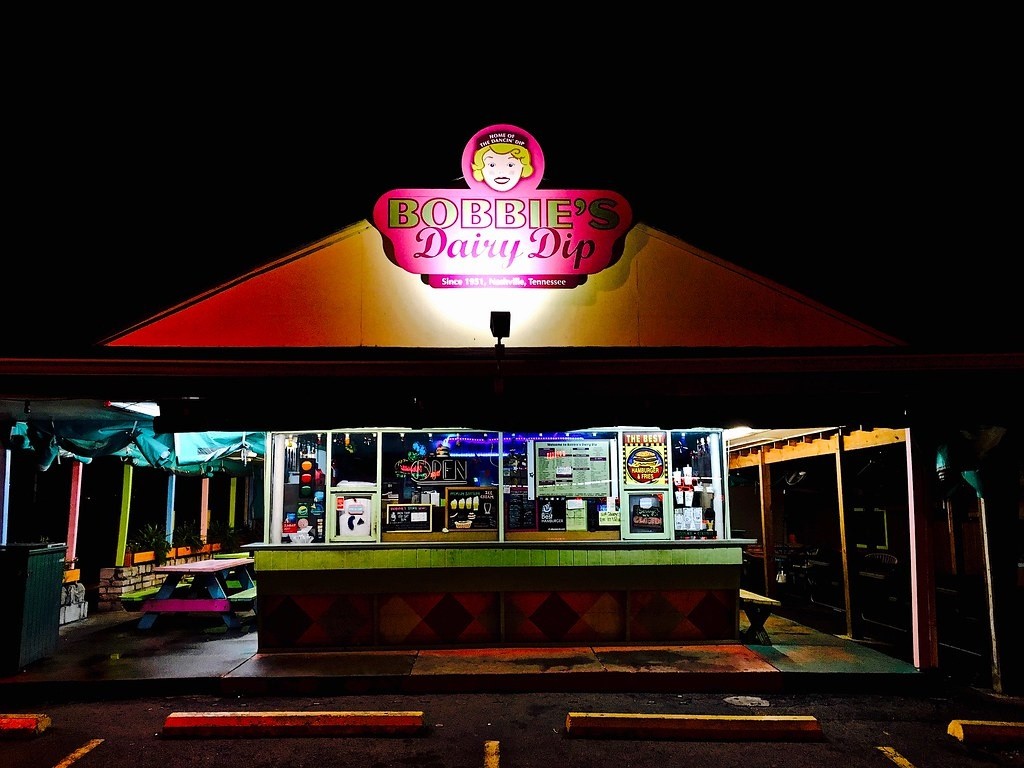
[138,559,255,628]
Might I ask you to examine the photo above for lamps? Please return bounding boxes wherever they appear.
[490,310,511,347]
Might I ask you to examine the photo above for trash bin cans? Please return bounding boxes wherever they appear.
[0,543,72,675]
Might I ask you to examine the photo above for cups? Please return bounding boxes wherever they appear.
[673,467,694,507]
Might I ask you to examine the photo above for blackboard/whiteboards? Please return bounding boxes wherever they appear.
[386,503,434,533]
[504,487,540,532]
[445,486,499,531]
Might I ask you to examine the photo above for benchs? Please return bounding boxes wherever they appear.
[739,589,781,646]
[116,571,257,630]
